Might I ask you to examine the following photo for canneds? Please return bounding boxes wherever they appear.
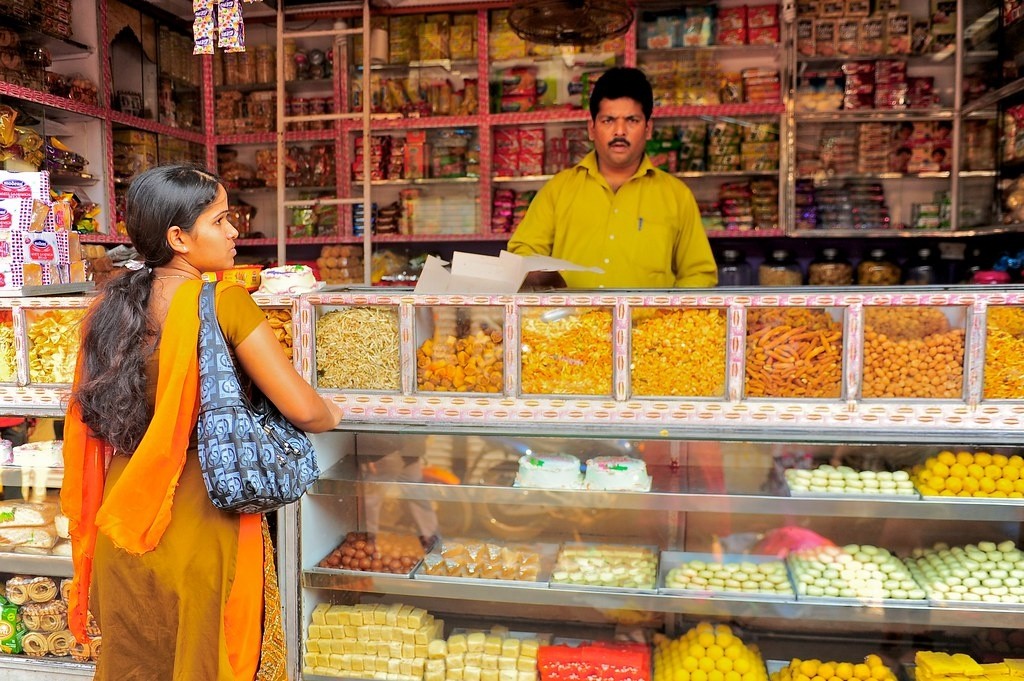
[157,25,297,87]
[285,95,336,131]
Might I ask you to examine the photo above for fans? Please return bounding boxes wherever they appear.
[506,0,634,48]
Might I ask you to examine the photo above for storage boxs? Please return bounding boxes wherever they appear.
[792,1,1023,227]
[217,11,484,241]
[0,0,212,243]
[480,5,783,231]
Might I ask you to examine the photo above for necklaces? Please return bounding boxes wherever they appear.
[150,275,192,283]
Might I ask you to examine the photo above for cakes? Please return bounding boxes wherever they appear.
[512,452,582,490]
[581,454,657,494]
[0,437,15,466]
[12,439,65,467]
[258,264,317,294]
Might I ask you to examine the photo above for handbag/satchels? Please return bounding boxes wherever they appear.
[197,281,319,514]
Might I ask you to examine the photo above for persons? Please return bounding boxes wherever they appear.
[59,164,342,681]
[506,67,718,321]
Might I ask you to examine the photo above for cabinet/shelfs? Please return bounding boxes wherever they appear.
[102,0,215,243]
[0,0,115,245]
[954,0,1024,235]
[787,0,960,237]
[481,3,630,241]
[205,5,346,246]
[629,1,791,238]
[0,289,1024,681]
[339,6,487,242]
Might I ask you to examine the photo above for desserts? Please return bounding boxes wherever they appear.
[302,602,1024,681]
[0,574,102,662]
[315,530,1024,604]
[782,448,1024,501]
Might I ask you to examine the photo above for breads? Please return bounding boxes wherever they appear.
[78,244,129,287]
[0,494,74,556]
